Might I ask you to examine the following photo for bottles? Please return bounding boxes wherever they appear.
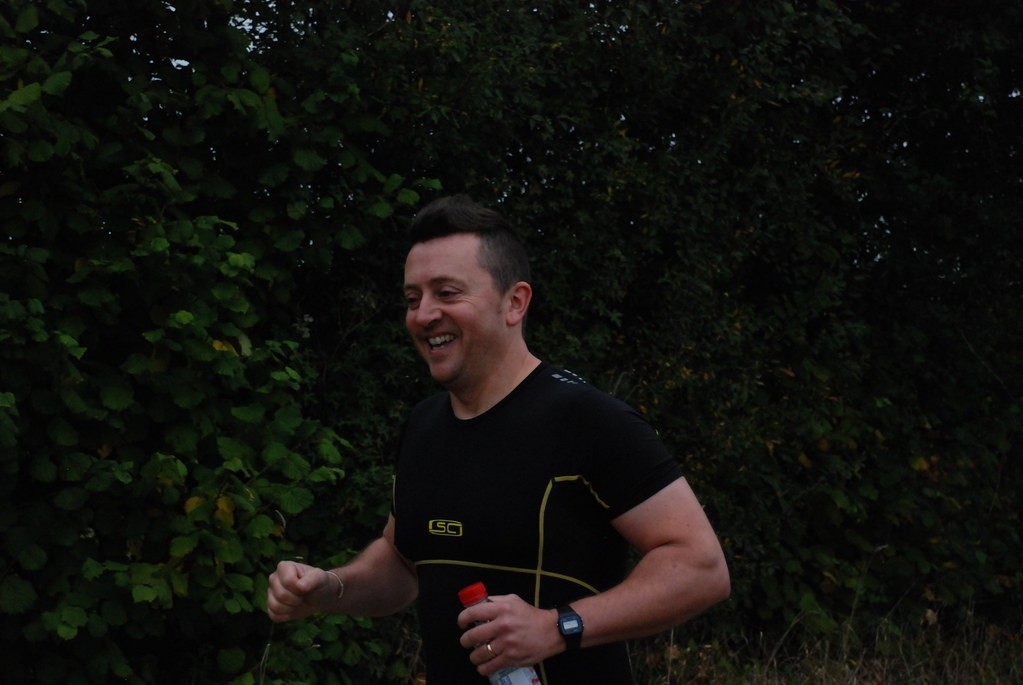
[458,580,541,685]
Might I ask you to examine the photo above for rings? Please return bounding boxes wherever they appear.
[486,642,497,657]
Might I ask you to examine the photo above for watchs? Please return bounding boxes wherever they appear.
[555,605,585,651]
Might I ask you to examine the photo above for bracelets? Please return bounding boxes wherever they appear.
[325,571,344,615]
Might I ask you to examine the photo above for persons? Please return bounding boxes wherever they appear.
[268,194,729,685]
